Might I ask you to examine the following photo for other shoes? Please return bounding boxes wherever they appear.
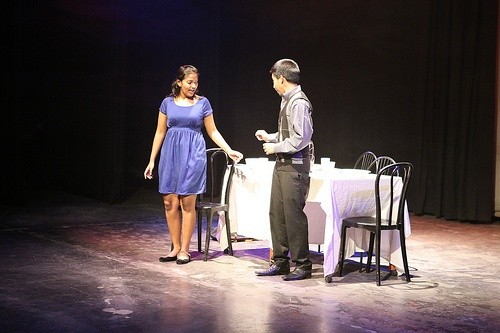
[159,249,179,262]
[176,250,191,264]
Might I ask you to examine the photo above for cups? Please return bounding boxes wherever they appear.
[321,158,336,172]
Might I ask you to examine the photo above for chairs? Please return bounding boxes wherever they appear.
[354,152,378,174]
[367,156,399,177]
[339,162,414,286]
[171,148,233,261]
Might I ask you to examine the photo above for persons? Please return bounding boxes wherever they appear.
[255,59,313,281]
[144,65,243,264]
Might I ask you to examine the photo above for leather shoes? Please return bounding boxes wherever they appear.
[282,268,311,280]
[255,264,290,276]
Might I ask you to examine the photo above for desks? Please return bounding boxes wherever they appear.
[216,164,412,282]
